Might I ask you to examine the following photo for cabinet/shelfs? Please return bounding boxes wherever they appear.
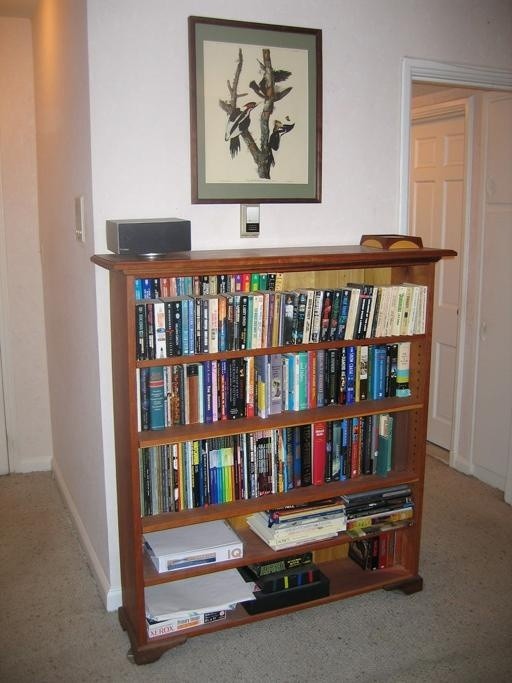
[88,243,459,666]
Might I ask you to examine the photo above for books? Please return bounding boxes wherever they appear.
[138,413,416,638]
[135,271,427,361]
[136,340,413,432]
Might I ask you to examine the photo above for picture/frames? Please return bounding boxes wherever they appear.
[187,16,321,205]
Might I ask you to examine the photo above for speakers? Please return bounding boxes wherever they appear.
[105,217,192,257]
